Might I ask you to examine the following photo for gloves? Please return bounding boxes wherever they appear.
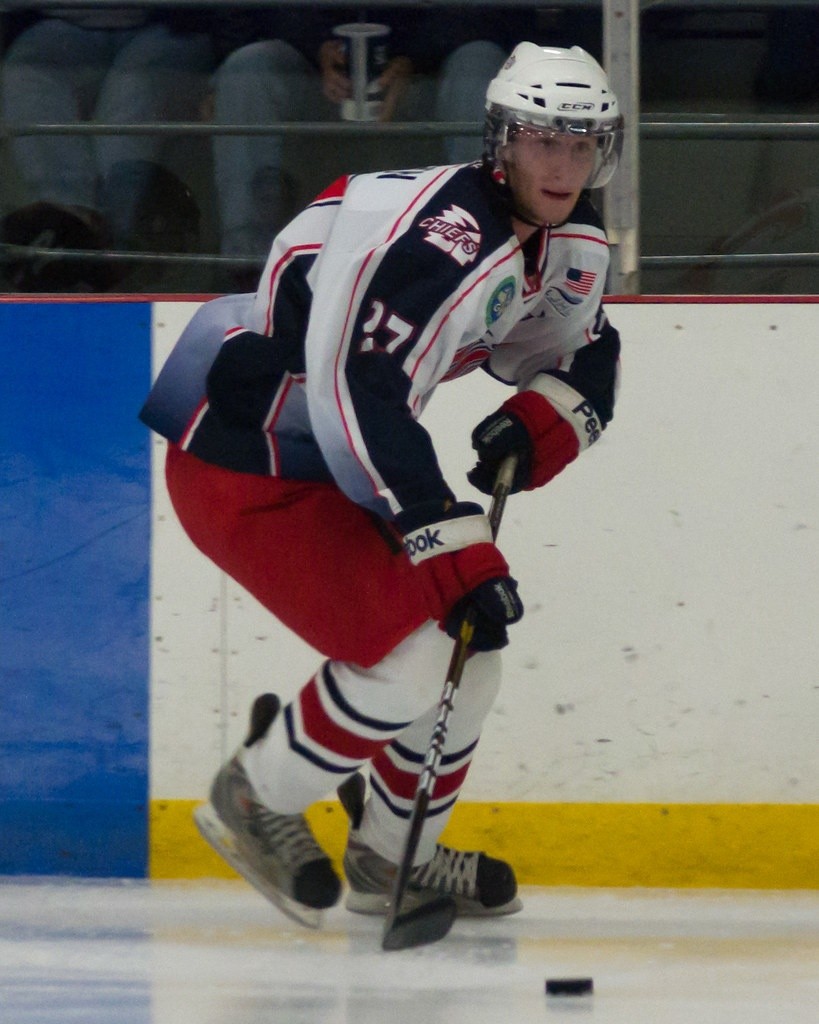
[389,500,524,654]
[467,366,610,496]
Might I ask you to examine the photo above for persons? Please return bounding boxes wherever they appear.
[140,40,634,940]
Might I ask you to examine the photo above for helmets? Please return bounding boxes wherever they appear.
[483,38,621,191]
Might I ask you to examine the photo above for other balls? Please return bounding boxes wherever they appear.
[544,977,592,996]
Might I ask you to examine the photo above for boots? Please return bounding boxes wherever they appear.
[330,771,530,918]
[193,692,337,925]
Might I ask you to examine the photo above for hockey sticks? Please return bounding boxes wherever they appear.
[380,436,524,952]
